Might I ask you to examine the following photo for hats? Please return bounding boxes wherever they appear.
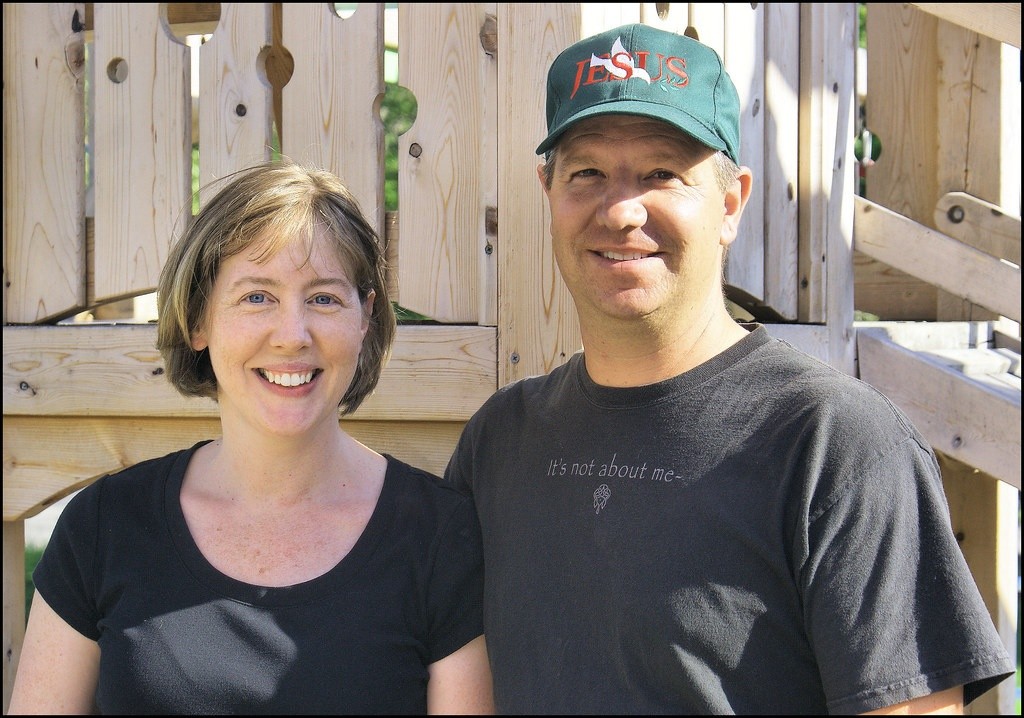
[536,23,740,167]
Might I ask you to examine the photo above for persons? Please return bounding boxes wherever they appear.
[8,153,496,716]
[442,25,1016,715]
